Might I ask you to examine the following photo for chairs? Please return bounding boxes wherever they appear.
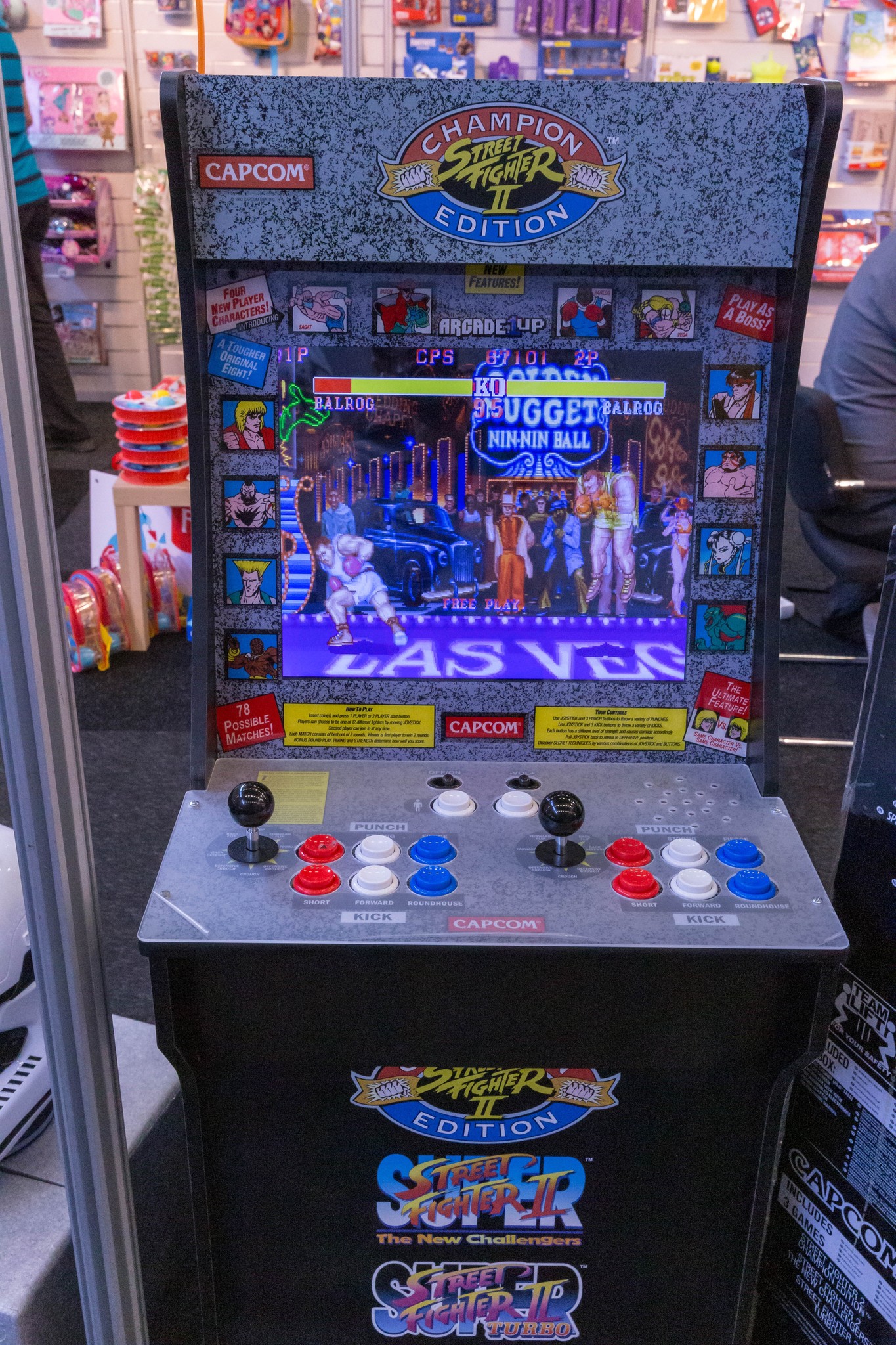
[776,385,896,644]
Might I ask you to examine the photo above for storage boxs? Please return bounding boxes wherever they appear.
[18,57,126,151]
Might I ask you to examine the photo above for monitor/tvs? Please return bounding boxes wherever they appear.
[279,348,705,678]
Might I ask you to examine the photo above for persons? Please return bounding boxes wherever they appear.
[811,229,896,551]
[0,19,99,453]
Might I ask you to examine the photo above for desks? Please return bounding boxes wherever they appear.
[115,477,192,652]
